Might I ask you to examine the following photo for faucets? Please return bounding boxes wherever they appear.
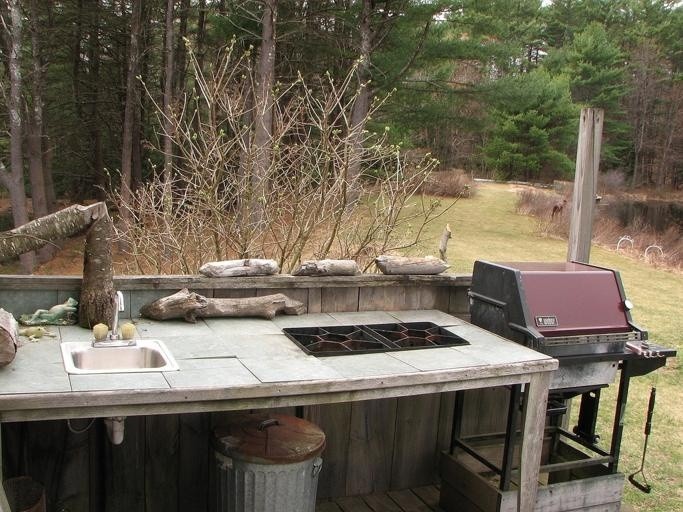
[110,293,125,341]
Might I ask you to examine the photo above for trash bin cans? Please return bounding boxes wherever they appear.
[209,414,327,512]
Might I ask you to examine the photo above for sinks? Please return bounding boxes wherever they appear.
[60,340,181,372]
